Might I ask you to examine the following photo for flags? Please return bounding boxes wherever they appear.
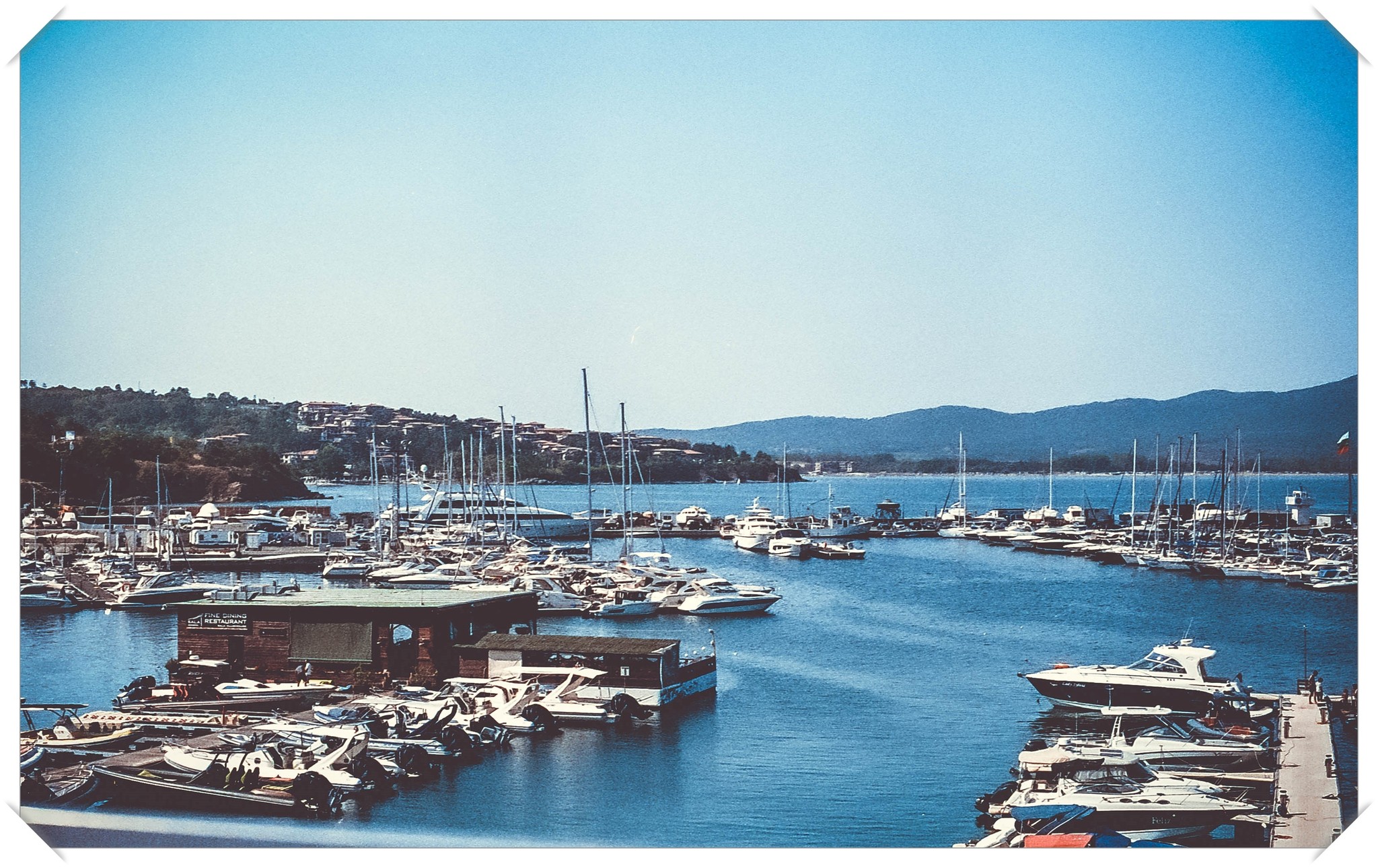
[1337,431,1350,455]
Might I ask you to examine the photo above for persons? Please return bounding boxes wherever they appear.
[1324,696,1333,718]
[1340,688,1349,711]
[1314,677,1324,701]
[1308,670,1318,704]
[295,664,308,687]
[303,659,312,684]
[1351,683,1358,708]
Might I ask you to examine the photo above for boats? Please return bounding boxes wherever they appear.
[21,363,1356,848]
